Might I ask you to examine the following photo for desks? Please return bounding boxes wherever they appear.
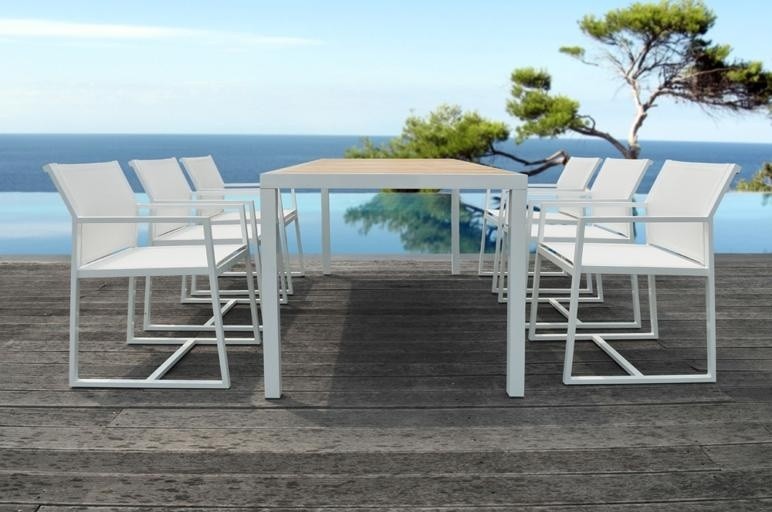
[255,157,528,398]
